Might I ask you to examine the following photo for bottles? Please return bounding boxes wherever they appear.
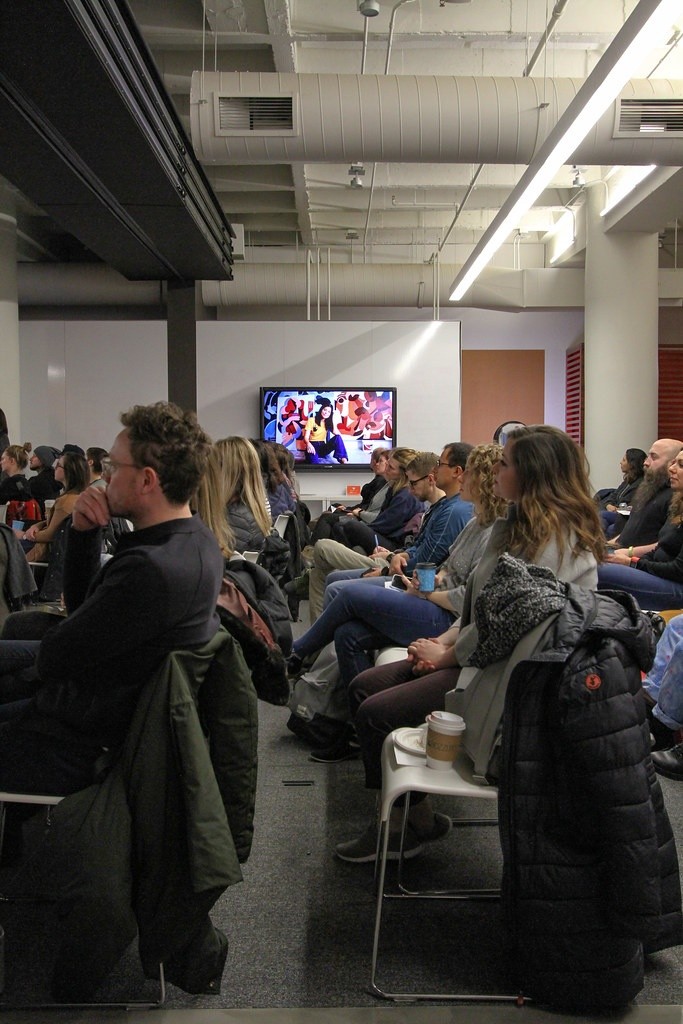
[619,503,627,511]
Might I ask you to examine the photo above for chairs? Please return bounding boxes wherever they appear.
[0,513,531,1009]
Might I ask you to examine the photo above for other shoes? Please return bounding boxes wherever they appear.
[286,656,302,676]
[311,731,363,764]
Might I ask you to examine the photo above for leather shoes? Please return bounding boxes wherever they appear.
[651,742,683,781]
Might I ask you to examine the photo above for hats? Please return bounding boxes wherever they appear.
[34,445,62,466]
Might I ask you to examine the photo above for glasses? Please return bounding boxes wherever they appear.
[436,460,457,467]
[55,462,64,469]
[100,456,142,476]
[410,475,428,486]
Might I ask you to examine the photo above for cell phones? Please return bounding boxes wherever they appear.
[391,573,412,591]
[339,515,358,523]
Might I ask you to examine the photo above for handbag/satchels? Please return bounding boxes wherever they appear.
[4,500,41,527]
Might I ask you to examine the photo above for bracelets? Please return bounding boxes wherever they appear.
[630,557,639,567]
[418,591,426,599]
[23,535,26,540]
[358,509,363,516]
[629,547,632,557]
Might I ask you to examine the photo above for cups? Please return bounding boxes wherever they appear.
[45,500,56,517]
[416,562,437,591]
[12,521,24,531]
[425,711,465,771]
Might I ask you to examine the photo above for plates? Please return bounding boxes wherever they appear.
[395,728,426,755]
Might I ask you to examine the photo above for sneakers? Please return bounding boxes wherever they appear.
[283,575,309,597]
[334,821,422,863]
[407,812,451,842]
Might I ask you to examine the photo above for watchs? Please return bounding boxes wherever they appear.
[381,566,389,576]
[386,554,393,563]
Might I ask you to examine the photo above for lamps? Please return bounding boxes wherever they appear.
[446,0,683,301]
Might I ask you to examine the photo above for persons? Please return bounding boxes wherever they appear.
[304,404,348,464]
[249,437,300,519]
[0,445,235,705]
[0,401,224,865]
[335,425,609,862]
[287,443,511,763]
[217,435,271,552]
[598,442,683,610]
[643,612,683,782]
[0,442,110,562]
[289,442,476,716]
[597,439,683,557]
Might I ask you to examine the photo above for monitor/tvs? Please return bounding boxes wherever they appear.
[260,387,398,468]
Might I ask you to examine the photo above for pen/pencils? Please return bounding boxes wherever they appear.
[375,534,379,549]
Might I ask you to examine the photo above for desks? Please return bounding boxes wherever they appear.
[299,494,363,513]
